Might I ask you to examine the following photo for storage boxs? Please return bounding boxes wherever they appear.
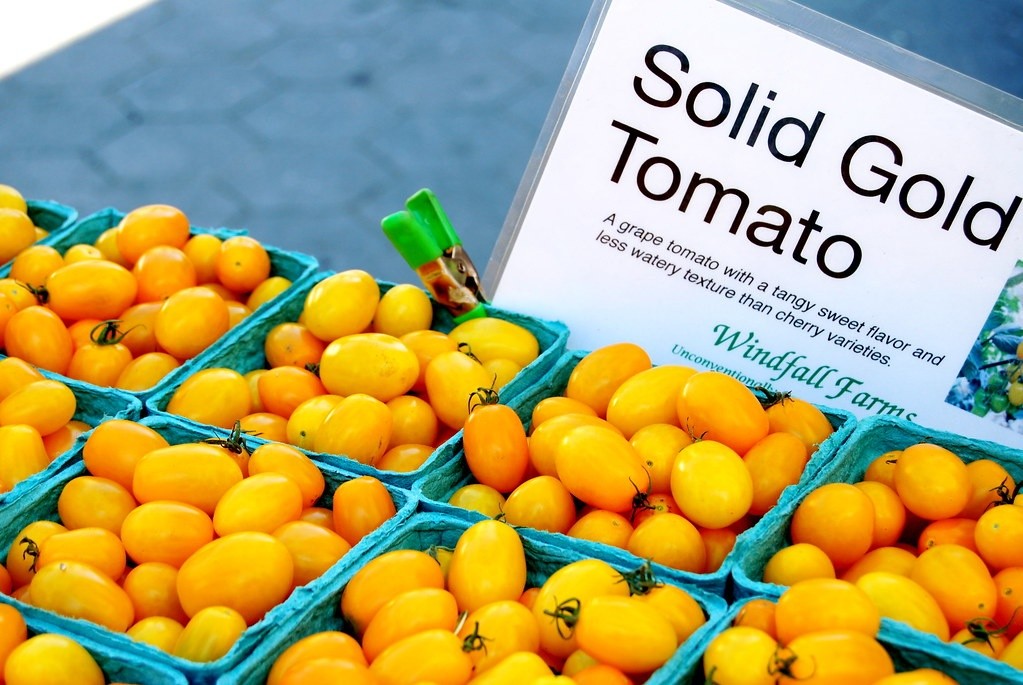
[2,176,1023,685]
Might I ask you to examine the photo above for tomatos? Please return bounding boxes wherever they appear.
[0,180,1023,685]
